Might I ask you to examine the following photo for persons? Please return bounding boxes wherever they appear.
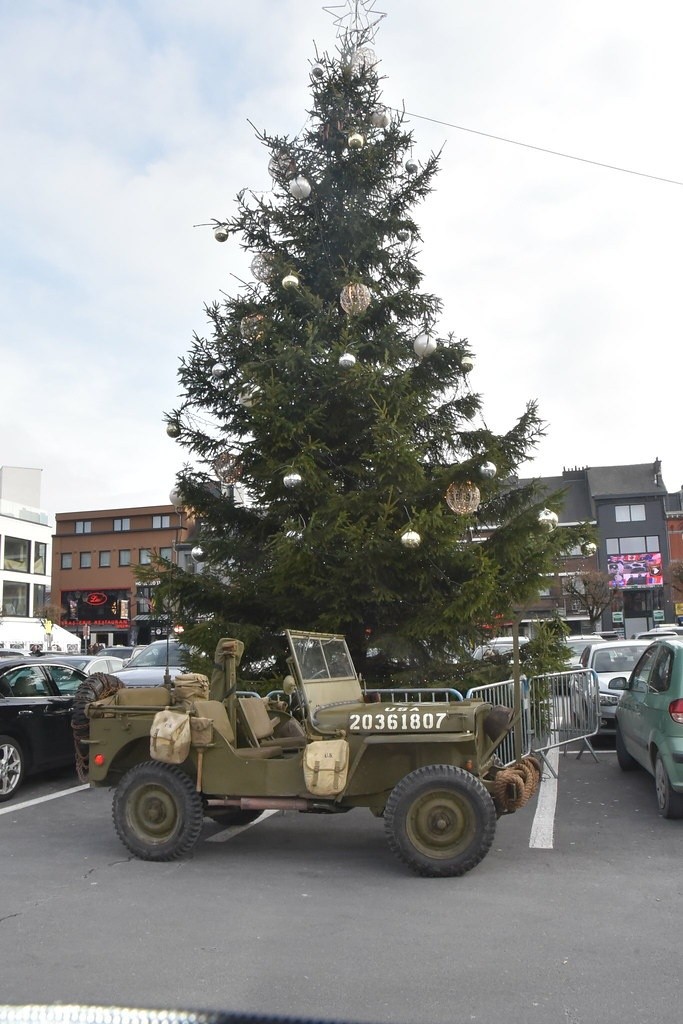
[87,642,104,656]
[31,644,41,656]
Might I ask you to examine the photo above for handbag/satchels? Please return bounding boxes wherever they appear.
[150,711,191,764]
[303,739,349,796]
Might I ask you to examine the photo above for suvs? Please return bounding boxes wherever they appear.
[107,637,205,689]
[71,630,543,877]
[544,635,609,695]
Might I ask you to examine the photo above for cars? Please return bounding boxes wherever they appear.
[570,639,656,743]
[96,645,158,667]
[472,637,530,659]
[608,637,683,818]
[649,624,683,636]
[631,631,678,642]
[0,659,88,803]
[42,655,128,693]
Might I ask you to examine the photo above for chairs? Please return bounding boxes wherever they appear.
[192,698,284,759]
[232,689,308,754]
[11,676,37,697]
[595,652,613,673]
